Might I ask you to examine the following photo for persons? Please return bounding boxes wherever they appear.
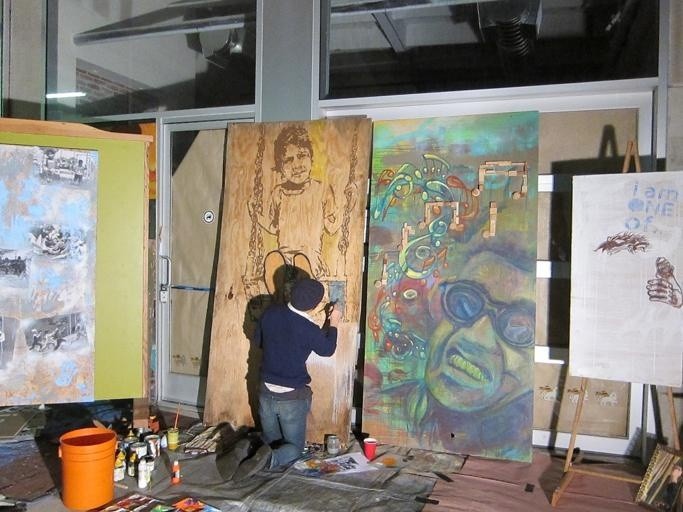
[216,277,339,483]
[382,232,535,463]
[243,127,359,310]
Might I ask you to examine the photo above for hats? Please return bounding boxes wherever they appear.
[290,279,325,310]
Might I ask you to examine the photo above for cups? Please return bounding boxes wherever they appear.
[363,437,377,458]
[167,427,179,450]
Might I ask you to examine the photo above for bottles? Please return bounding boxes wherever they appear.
[114,451,137,483]
[138,459,151,491]
[117,433,125,442]
[117,442,125,451]
[326,435,339,454]
[171,461,179,484]
[125,429,161,459]
[143,455,154,471]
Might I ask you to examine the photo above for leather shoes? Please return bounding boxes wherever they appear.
[220,439,250,482]
[234,445,273,482]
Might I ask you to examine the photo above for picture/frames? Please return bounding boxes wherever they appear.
[634,443,683,512]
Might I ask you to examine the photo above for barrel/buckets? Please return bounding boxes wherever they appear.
[57,427,117,511]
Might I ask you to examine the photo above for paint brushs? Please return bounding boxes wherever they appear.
[317,298,340,314]
[174,402,181,429]
[367,450,390,464]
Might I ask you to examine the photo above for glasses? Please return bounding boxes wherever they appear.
[440,280,535,347]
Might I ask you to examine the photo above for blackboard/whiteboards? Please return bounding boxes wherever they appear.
[568,170,682,388]
